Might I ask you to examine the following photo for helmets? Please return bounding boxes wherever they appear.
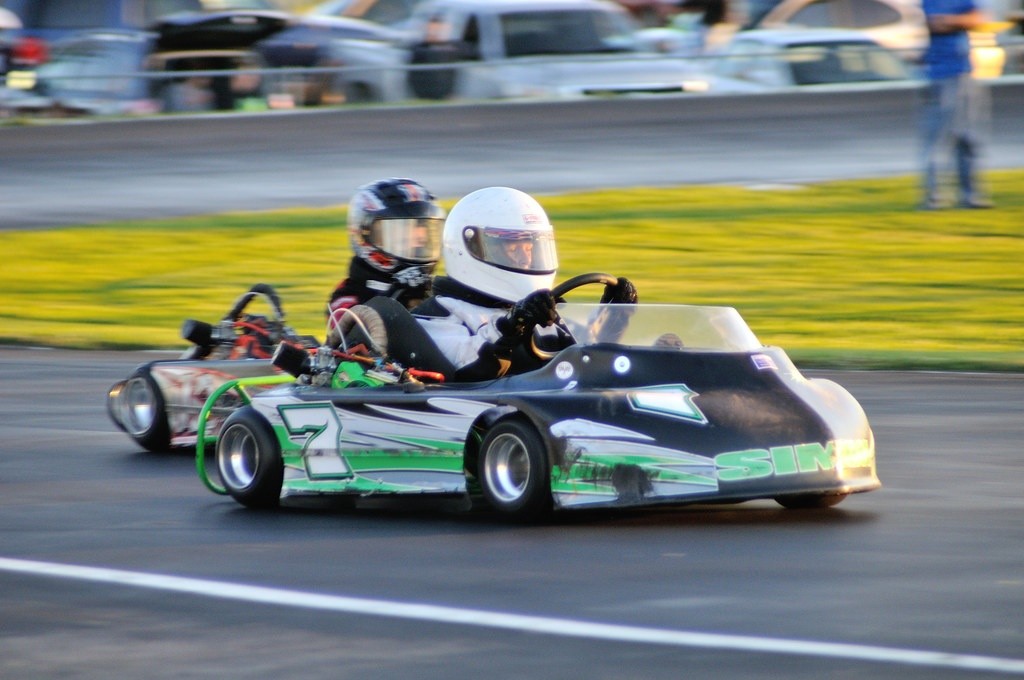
[346,179,447,286]
[441,187,558,305]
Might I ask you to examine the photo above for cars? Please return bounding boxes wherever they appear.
[0,1,1024,120]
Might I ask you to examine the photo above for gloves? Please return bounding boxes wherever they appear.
[496,290,559,343]
[389,269,433,293]
[601,278,639,323]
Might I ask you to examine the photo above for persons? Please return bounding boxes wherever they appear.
[0,31,52,102]
[405,15,484,99]
[306,57,347,106]
[223,61,271,112]
[915,1,994,209]
[410,185,640,382]
[163,65,216,113]
[324,178,446,362]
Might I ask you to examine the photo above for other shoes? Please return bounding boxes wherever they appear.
[959,197,996,207]
[918,196,952,208]
